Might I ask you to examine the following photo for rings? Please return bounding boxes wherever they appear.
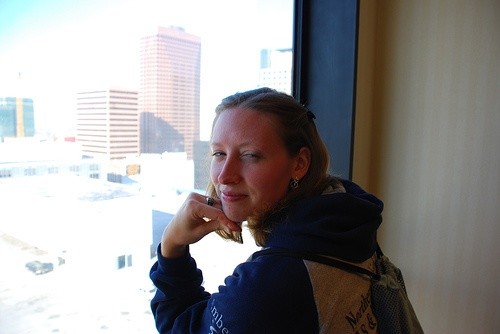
[206,196,214,206]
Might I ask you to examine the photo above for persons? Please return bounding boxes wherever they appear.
[150,87,424,334]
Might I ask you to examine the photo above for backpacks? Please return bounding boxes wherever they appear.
[247,240,425,334]
